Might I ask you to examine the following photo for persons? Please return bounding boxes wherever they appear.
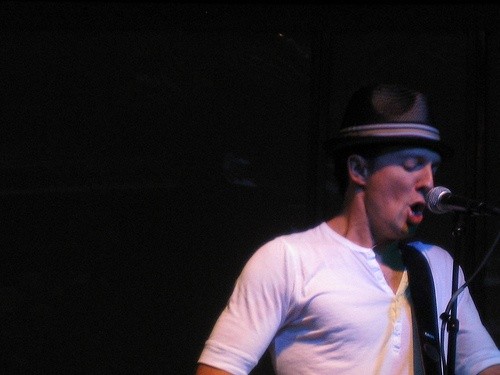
[192,87,500,375]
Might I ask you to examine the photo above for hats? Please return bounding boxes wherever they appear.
[321,80,452,158]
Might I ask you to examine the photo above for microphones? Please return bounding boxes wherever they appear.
[425,186,500,216]
